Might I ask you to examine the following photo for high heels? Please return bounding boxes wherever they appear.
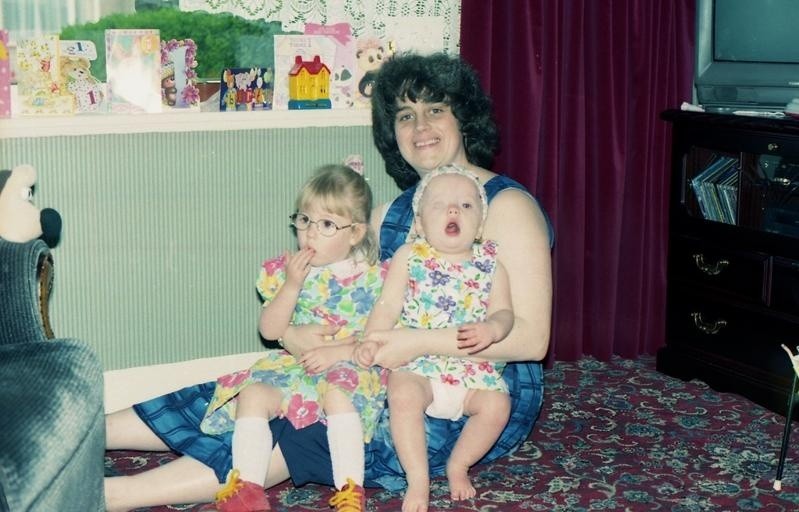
[198,468,272,512]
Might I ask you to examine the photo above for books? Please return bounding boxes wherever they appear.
[691,156,740,226]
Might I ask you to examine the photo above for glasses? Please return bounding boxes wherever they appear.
[288,212,360,238]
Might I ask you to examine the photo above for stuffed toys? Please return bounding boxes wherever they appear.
[0,165,61,244]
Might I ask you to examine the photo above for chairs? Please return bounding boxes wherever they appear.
[0,165,112,510]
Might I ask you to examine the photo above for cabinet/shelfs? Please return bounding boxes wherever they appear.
[657,103,795,418]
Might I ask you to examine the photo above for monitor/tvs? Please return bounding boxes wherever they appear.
[694,0,799,114]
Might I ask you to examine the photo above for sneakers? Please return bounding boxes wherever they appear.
[328,477,367,512]
